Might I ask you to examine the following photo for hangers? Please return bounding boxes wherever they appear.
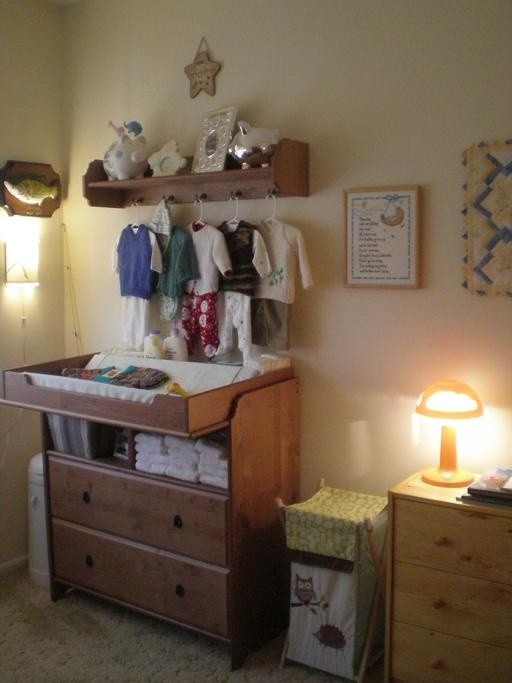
[226,197,244,227]
[258,192,282,230]
[128,202,145,232]
[190,199,209,229]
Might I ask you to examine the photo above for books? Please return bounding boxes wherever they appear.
[455,468,512,506]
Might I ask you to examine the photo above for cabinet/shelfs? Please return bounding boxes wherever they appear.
[0,349,300,671]
[387,464,512,683]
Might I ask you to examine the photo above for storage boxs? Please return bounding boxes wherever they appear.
[276,477,387,683]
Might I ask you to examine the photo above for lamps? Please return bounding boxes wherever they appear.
[413,377,484,488]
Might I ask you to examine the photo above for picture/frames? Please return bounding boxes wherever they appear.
[462,140,510,299]
[194,105,235,173]
[343,184,422,289]
[184,35,219,98]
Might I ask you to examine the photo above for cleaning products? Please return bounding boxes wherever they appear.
[149,339,161,358]
[144,329,163,359]
[163,324,189,361]
